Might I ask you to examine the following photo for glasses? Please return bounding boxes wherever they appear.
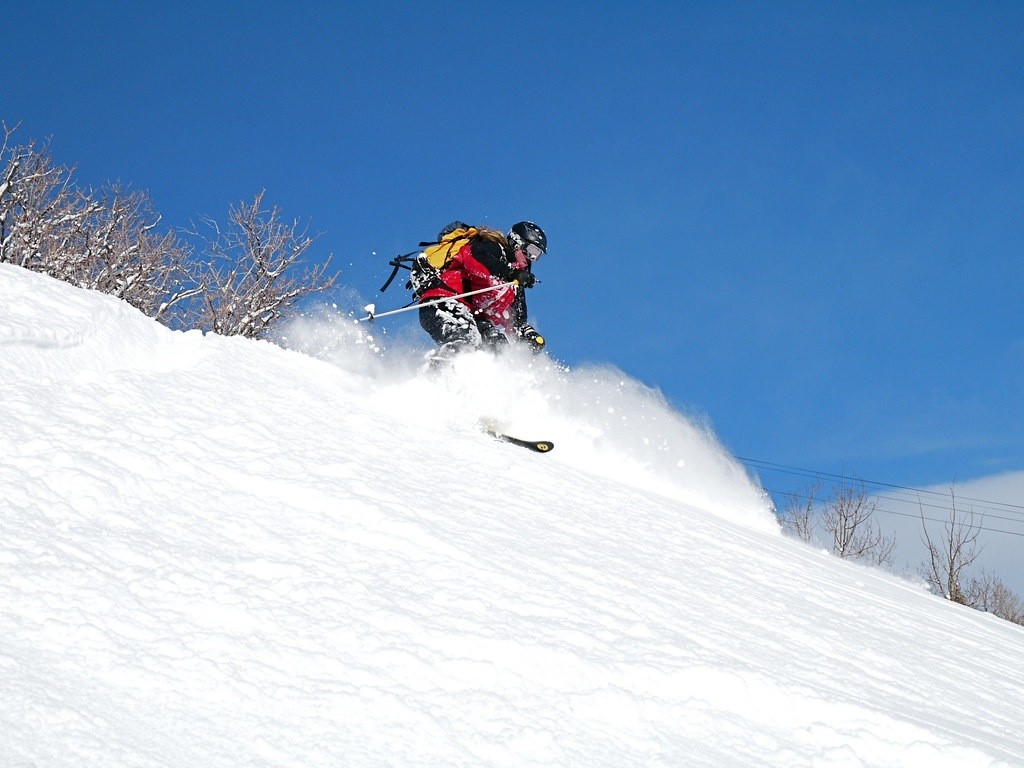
[521,242,542,261]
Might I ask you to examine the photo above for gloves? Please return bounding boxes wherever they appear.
[519,325,545,356]
[508,269,536,288]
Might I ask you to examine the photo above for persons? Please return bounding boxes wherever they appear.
[419,220,547,370]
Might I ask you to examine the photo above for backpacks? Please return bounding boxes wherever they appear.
[409,221,504,306]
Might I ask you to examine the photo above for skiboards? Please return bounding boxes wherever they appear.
[465,410,556,453]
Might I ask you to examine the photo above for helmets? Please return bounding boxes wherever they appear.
[507,221,547,255]
